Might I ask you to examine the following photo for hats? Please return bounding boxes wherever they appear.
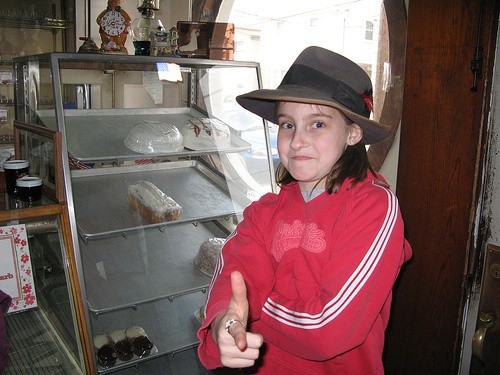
[235,45,393,146]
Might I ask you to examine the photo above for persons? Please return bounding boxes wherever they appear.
[195,46,412,375]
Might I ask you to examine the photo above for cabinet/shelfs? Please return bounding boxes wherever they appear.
[0,52,277,375]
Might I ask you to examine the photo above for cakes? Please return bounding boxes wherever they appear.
[195,237,226,276]
[180,118,230,151]
[125,121,183,154]
[93,326,153,369]
[128,181,181,224]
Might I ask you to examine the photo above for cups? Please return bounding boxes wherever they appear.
[4,160,29,200]
[17,177,42,202]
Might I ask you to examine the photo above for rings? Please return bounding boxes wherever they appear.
[225,318,246,334]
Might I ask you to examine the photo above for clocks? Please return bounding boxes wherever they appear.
[96,0,132,55]
